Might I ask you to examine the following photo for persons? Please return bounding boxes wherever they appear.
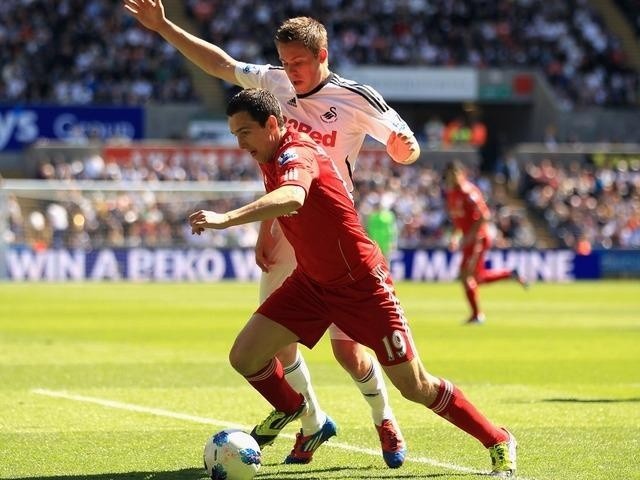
[443,158,530,325]
[189,87,518,477]
[124,1,421,467]
[1,0,639,251]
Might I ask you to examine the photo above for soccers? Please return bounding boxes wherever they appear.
[204,428,261,480]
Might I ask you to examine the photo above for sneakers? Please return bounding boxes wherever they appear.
[463,314,486,324]
[375,418,407,468]
[250,393,309,450]
[488,427,517,477]
[513,268,529,288]
[284,416,336,463]
